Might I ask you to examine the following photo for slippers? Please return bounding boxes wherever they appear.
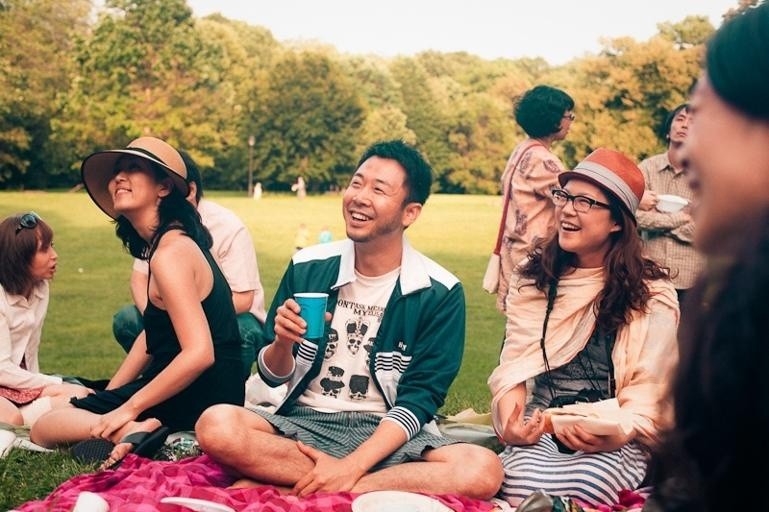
[102,424,169,472]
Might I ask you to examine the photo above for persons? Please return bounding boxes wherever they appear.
[194,139,504,501]
[296,223,309,250]
[29,135,245,472]
[0,212,96,430]
[113,151,265,385]
[638,0,768,511]
[291,176,307,202]
[252,180,262,203]
[319,224,331,245]
[495,84,658,316]
[487,146,681,512]
[637,103,706,313]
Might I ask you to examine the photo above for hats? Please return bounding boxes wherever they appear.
[80,136,190,219]
[558,146,645,228]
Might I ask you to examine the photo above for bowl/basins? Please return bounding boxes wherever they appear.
[657,195,689,213]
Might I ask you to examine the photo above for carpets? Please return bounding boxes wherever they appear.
[13,454,644,511]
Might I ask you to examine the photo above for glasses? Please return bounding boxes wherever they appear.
[549,189,611,214]
[562,114,576,121]
[15,213,38,234]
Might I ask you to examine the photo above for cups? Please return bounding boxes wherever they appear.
[293,293,328,339]
